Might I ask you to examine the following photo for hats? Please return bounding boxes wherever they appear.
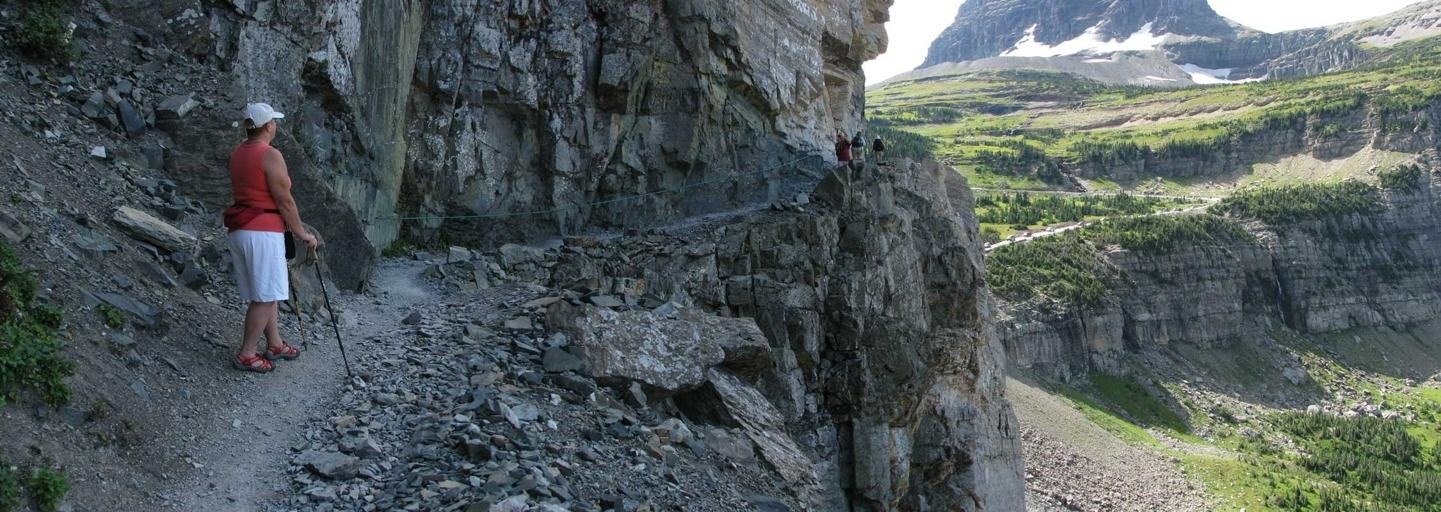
[243,103,286,131]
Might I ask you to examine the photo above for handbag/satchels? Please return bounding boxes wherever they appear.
[222,201,283,233]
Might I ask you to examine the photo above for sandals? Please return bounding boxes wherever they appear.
[264,341,302,361]
[235,355,277,373]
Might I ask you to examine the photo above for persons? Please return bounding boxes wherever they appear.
[222,102,322,376]
[836,133,853,161]
[872,136,885,164]
[852,131,867,160]
